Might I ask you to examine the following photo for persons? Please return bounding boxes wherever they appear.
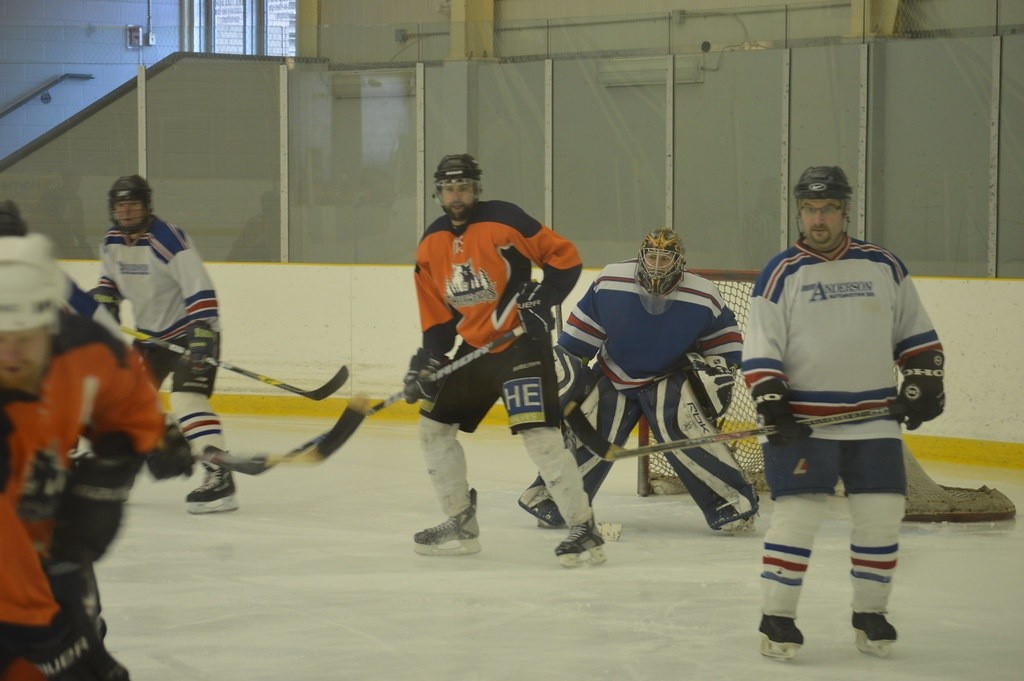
[516,227,759,534]
[86,174,238,515]
[0,200,193,483]
[742,165,946,662]
[403,153,608,569]
[0,236,163,681]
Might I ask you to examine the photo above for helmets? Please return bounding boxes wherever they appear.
[0,233,73,332]
[637,229,685,296]
[434,155,481,182]
[794,166,852,200]
[109,175,151,233]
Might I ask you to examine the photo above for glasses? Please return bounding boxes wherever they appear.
[799,204,844,217]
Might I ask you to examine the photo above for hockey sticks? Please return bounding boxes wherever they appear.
[190,387,371,470]
[214,322,522,474]
[119,324,349,402]
[561,398,907,461]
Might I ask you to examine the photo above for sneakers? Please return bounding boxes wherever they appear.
[186,462,238,514]
[758,614,803,658]
[413,488,482,555]
[852,612,897,657]
[719,513,756,533]
[555,514,607,568]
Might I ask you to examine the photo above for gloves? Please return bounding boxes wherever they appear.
[897,350,945,430]
[752,380,814,448]
[87,286,120,327]
[516,282,560,341]
[176,325,219,379]
[0,614,130,681]
[403,348,452,404]
